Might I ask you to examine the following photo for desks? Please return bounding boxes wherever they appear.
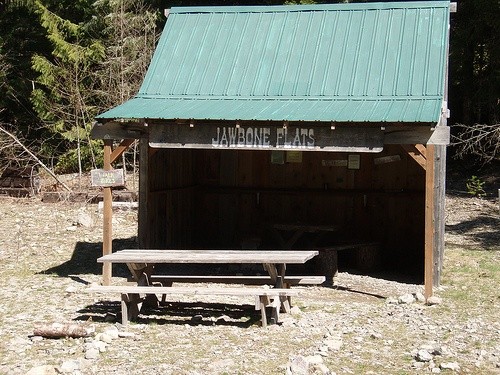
[96,249,319,322]
[265,219,344,249]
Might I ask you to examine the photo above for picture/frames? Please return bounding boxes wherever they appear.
[347,154,361,170]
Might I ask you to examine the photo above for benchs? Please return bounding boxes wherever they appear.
[310,240,384,275]
[236,231,263,249]
[88,284,302,330]
[127,274,326,310]
